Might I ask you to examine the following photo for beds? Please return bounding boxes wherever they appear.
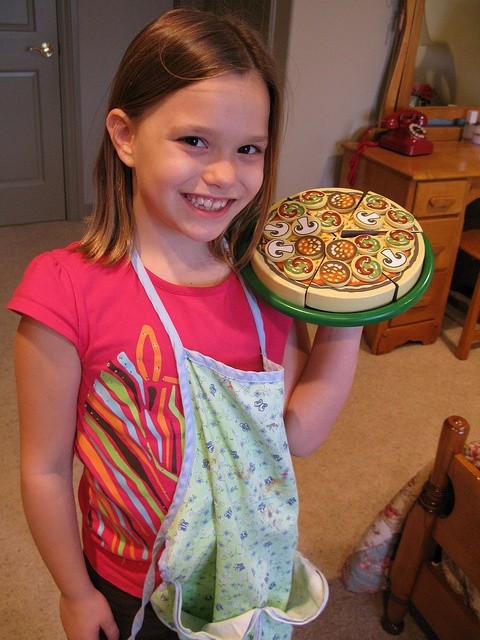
[383,417,480,640]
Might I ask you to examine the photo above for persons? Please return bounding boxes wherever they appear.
[15,7,364,635]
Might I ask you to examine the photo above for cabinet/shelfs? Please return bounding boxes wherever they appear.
[338,141,479,356]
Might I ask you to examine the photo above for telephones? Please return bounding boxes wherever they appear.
[347,109,434,189]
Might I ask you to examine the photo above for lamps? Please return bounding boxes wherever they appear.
[418,10,433,49]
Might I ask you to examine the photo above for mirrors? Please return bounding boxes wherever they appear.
[378,1,480,121]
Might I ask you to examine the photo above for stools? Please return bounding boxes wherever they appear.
[443,226,480,361]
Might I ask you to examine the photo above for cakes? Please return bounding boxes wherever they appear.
[251,187,425,314]
